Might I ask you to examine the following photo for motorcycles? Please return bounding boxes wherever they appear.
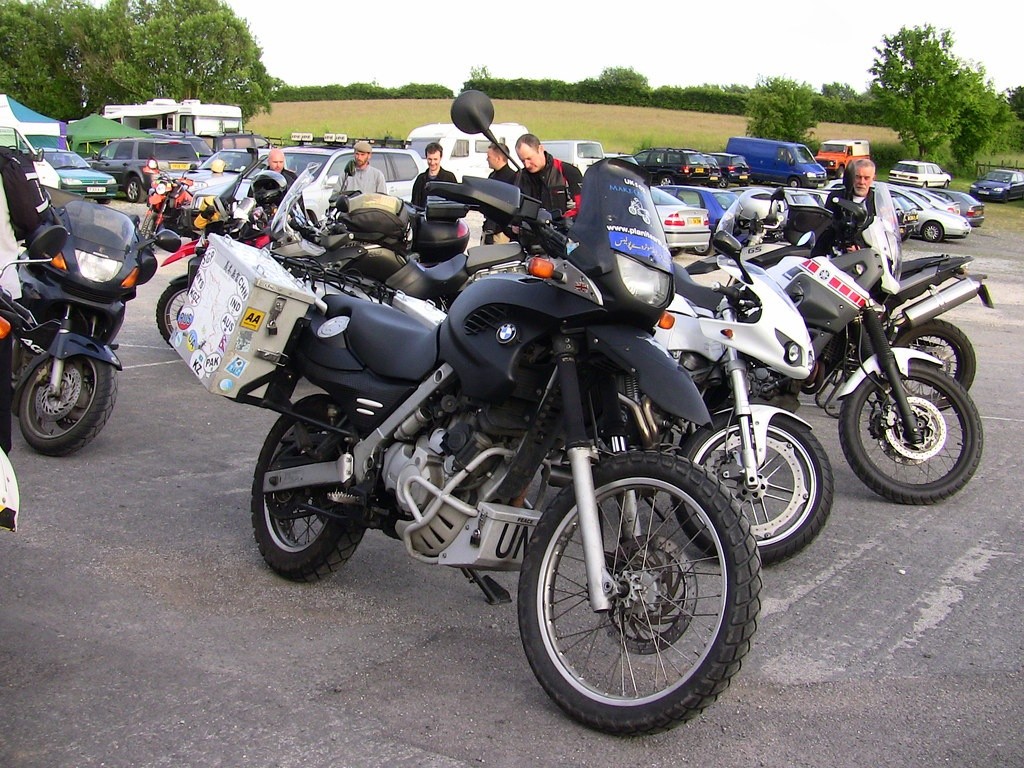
[0,184,182,457]
[155,90,994,735]
[0,225,69,534]
[140,157,198,240]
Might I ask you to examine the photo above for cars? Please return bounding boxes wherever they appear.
[888,160,952,189]
[22,148,119,205]
[702,153,722,188]
[709,153,752,188]
[648,169,1024,258]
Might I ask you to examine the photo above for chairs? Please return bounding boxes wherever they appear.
[994,173,1002,180]
[230,157,245,169]
[53,160,64,168]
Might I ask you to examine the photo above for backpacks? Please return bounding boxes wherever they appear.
[336,190,412,252]
[0,145,51,232]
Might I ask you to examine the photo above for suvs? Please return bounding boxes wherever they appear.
[604,152,640,167]
[82,128,428,224]
[633,147,712,186]
[814,139,871,179]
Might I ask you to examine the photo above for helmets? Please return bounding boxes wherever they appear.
[252,170,288,207]
[211,159,226,173]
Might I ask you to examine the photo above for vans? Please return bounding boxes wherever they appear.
[539,141,605,178]
[104,98,244,135]
[0,126,61,190]
[402,123,530,183]
[727,137,827,188]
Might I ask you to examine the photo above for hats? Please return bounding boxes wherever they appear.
[353,142,372,153]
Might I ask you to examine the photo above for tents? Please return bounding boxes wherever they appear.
[1,93,68,154]
[64,114,151,161]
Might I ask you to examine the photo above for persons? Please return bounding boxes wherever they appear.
[501,134,584,256]
[480,143,518,247]
[412,143,458,209]
[246,148,307,226]
[330,140,388,211]
[823,158,886,259]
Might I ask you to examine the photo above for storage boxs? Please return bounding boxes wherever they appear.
[167,233,316,405]
[391,289,447,330]
